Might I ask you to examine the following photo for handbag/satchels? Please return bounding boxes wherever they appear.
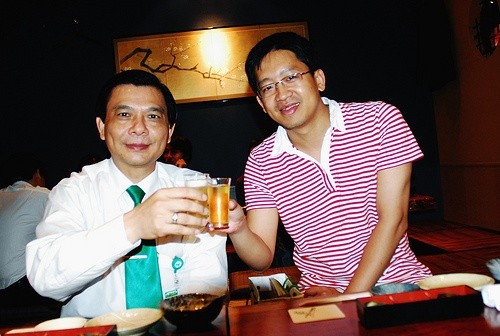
[245,273,304,308]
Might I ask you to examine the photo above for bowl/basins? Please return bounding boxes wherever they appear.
[487,258,500,281]
[160,292,224,328]
[370,283,419,296]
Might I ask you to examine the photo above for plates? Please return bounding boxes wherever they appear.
[34,316,89,331]
[419,273,496,290]
[84,307,163,336]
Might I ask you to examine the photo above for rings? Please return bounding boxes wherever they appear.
[171,212,178,224]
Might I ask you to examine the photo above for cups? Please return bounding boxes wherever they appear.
[183,173,211,233]
[208,178,231,231]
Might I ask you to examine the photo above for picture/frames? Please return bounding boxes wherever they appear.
[115,22,309,105]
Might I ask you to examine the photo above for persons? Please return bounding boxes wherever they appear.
[161,134,191,169]
[0,153,61,327]
[205,32,434,299]
[24,68,229,318]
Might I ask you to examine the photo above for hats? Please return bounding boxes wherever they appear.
[165,134,192,164]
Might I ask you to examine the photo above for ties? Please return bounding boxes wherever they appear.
[125,185,164,309]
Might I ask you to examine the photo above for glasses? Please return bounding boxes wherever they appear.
[257,69,311,98]
